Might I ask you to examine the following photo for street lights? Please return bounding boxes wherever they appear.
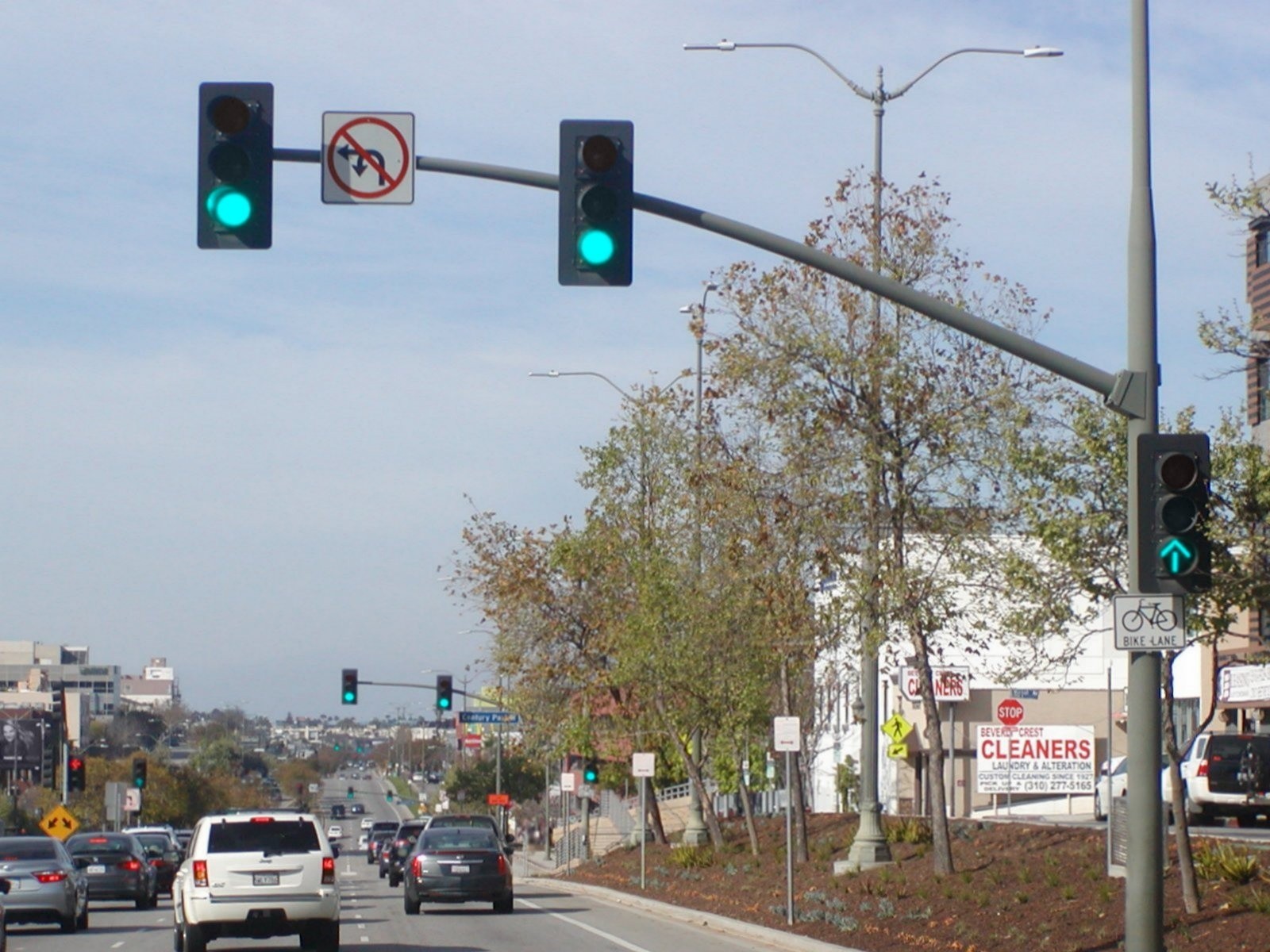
[419,573,503,831]
[0,702,42,781]
[75,699,245,772]
[682,40,1057,877]
[534,363,723,593]
[34,717,50,786]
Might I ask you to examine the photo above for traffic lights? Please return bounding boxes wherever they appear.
[340,668,357,706]
[386,790,393,802]
[346,787,354,800]
[434,675,452,710]
[583,764,597,784]
[131,757,146,788]
[557,116,635,288]
[1132,429,1214,596]
[194,82,275,251]
[64,755,84,791]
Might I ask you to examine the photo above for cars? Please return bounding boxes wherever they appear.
[170,828,193,860]
[1096,754,1171,819]
[328,802,514,915]
[62,832,158,911]
[0,837,90,934]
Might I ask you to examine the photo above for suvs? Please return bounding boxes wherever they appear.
[172,810,338,952]
[1161,731,1270,832]
[123,825,179,897]
